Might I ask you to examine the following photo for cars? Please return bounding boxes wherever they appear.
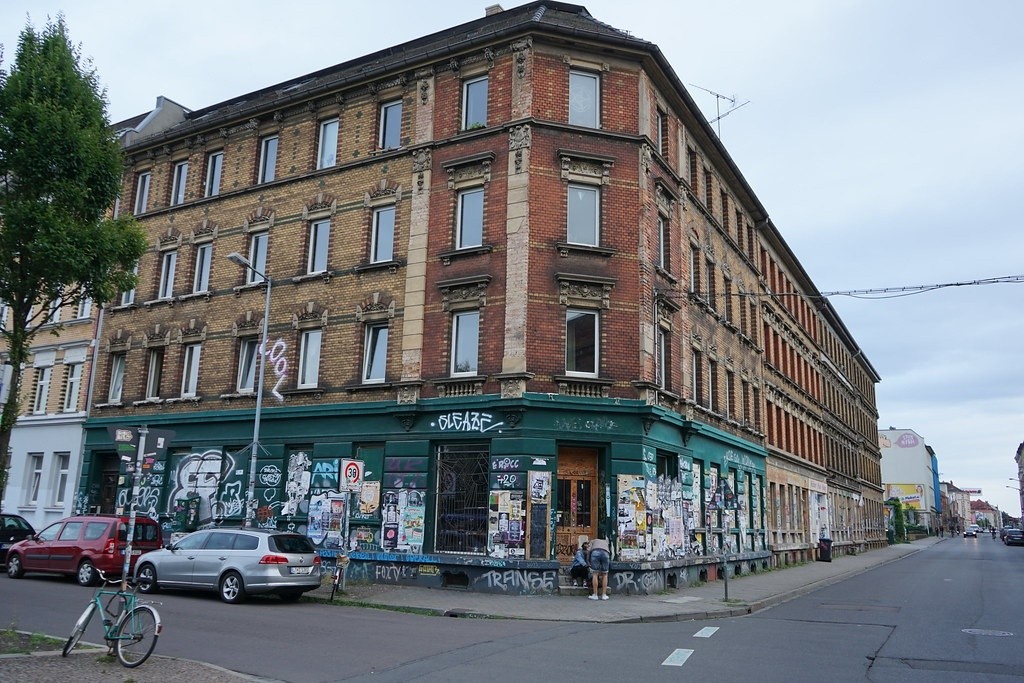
[1000,525,1024,546]
[439,514,487,552]
[6,513,164,586]
[963,524,983,538]
[0,512,37,564]
[132,528,322,604]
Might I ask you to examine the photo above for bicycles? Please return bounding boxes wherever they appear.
[330,546,357,600]
[61,564,163,668]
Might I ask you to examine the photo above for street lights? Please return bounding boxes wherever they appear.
[227,252,271,529]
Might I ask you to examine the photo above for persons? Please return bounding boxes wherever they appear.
[957,525,960,533]
[587,539,610,600]
[916,486,924,507]
[988,524,997,538]
[935,527,943,538]
[570,542,591,587]
[949,526,955,535]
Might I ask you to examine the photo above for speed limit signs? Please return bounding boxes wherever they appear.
[442,472,457,498]
[339,458,364,493]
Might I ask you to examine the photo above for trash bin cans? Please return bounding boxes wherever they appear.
[819,538,834,562]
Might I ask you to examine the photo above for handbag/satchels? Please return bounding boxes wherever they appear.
[588,578,602,588]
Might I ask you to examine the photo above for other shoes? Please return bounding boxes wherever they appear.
[573,580,578,586]
[588,594,598,600]
[583,584,588,589]
[602,594,609,600]
